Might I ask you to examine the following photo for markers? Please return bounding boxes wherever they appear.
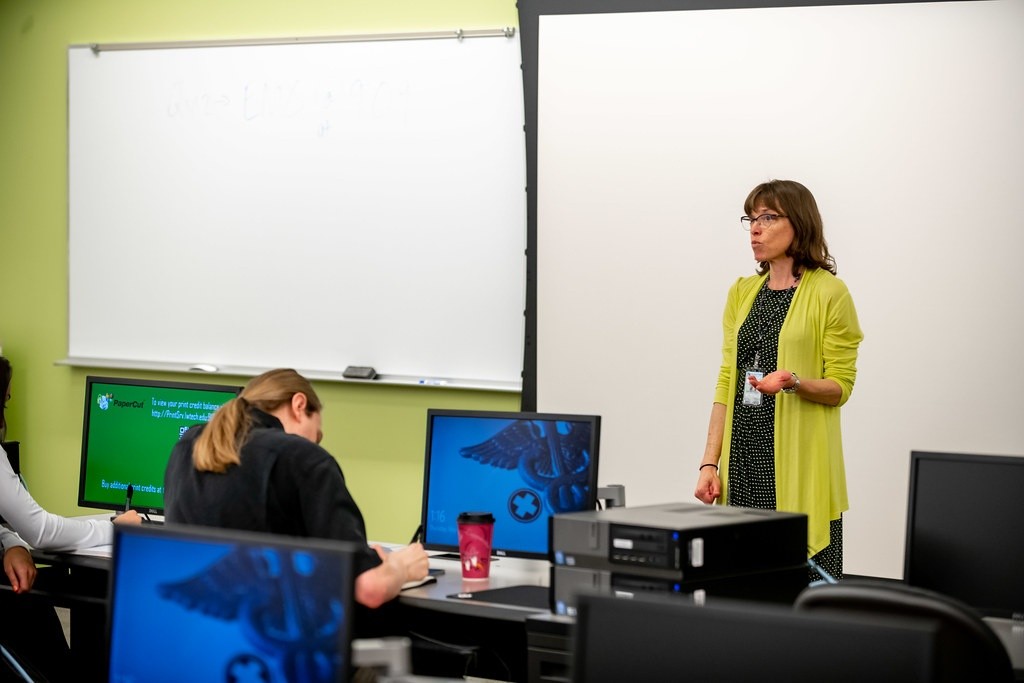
[418,379,448,386]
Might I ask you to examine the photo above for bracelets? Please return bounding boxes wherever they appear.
[700,463,718,471]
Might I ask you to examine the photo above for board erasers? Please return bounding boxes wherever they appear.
[189,364,219,372]
[342,366,377,380]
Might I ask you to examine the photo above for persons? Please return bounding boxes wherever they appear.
[694,179,864,587]
[165,370,429,607]
[0,356,142,683]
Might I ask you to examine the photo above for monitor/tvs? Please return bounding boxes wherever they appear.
[102,522,357,683]
[77,377,246,517]
[903,450,1024,621]
[571,587,938,683]
[419,408,601,560]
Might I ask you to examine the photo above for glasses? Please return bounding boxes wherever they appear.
[741,214,789,231]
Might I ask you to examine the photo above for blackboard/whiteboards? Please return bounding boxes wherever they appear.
[55,28,526,393]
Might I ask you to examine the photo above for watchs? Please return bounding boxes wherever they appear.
[781,374,800,395]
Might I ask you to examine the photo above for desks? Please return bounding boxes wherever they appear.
[0,539,555,683]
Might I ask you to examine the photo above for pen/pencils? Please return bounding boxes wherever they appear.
[124,485,133,512]
[408,525,424,544]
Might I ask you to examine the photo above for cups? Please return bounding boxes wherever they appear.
[457,512,495,582]
